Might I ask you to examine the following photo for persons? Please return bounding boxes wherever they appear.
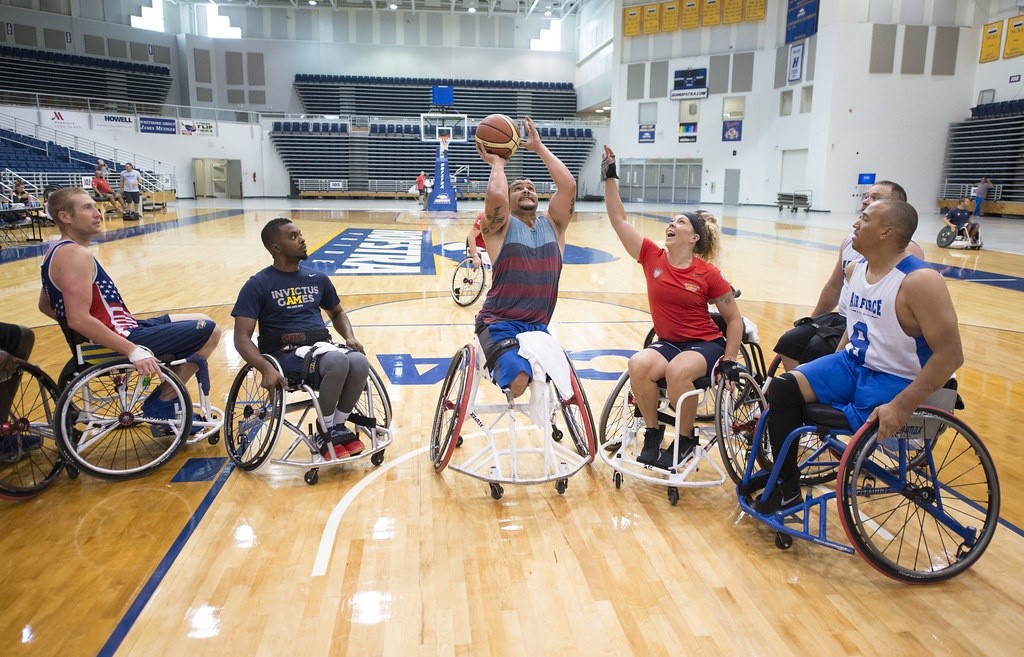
[9,182,29,206]
[774,181,924,372]
[943,197,980,240]
[476,117,576,399]
[230,218,369,467]
[92,171,127,213]
[602,144,743,466]
[750,198,964,516]
[120,163,143,217]
[409,171,435,205]
[38,188,221,434]
[98,160,111,179]
[0,323,43,464]
[972,176,992,217]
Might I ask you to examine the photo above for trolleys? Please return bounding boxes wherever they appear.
[774,189,813,213]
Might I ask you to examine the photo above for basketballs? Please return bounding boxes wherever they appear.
[475,113,521,159]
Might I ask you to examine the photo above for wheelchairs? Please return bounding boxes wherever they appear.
[596,314,758,509]
[936,224,985,249]
[737,366,1002,584]
[450,234,495,307]
[428,341,600,498]
[0,354,72,498]
[52,321,228,481]
[754,312,960,484]
[224,330,396,485]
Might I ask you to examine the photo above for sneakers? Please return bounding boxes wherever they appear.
[657,434,693,467]
[0,434,44,459]
[763,481,803,514]
[140,385,204,434]
[315,427,350,461]
[641,425,664,460]
[332,423,365,455]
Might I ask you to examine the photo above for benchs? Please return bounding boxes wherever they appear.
[92,196,127,222]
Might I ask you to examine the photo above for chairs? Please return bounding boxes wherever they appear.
[0,217,32,245]
[0,128,160,197]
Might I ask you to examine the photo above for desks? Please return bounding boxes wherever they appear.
[0,207,43,242]
